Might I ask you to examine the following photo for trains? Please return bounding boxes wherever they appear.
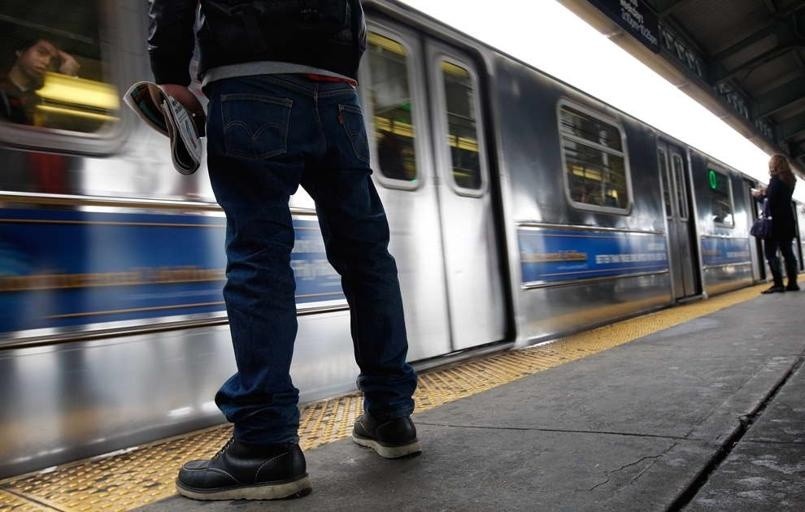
[0,0,805,479]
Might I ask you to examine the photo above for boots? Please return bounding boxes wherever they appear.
[785,259,799,290]
[763,260,784,293]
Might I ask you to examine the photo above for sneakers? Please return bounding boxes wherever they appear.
[175,435,312,501]
[353,413,422,460]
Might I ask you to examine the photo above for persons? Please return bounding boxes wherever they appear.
[150,0,421,500]
[753,154,801,294]
[0,35,82,129]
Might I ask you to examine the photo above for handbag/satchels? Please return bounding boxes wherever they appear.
[750,198,775,241]
